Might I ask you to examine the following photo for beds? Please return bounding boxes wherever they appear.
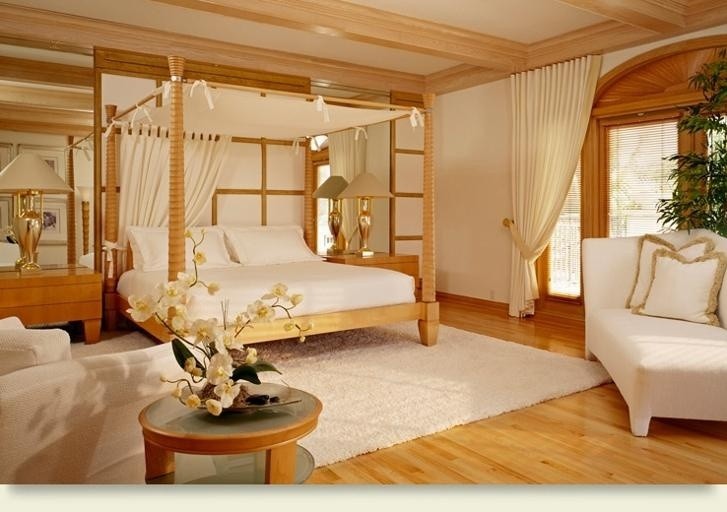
[103,56,439,361]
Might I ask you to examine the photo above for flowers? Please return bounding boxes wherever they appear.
[125,225,315,418]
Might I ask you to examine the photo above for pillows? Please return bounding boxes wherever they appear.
[220,225,327,263]
[624,234,715,309]
[633,247,726,327]
[126,225,242,271]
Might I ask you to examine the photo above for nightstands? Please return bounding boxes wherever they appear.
[319,252,420,301]
[0,262,105,344]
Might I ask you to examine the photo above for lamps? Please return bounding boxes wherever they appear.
[311,176,349,253]
[337,175,395,257]
[0,152,76,273]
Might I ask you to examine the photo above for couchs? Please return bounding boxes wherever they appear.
[0,316,211,484]
[581,227,726,436]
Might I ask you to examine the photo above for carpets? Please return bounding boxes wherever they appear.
[67,319,614,471]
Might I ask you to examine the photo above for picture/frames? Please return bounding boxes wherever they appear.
[18,192,68,245]
[0,193,17,242]
[17,144,69,199]
[0,142,14,171]
